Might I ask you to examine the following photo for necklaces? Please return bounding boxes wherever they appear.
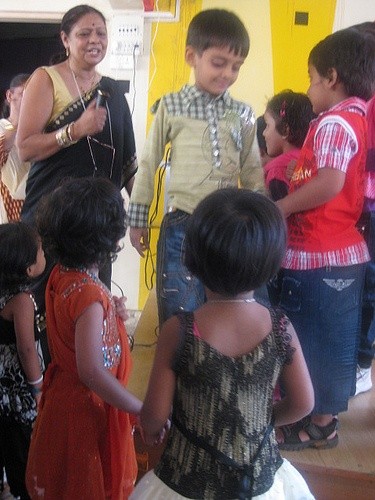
[206,299,257,303]
[66,60,97,100]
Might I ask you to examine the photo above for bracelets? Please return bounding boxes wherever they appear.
[139,427,165,446]
[55,121,78,148]
[26,375,44,393]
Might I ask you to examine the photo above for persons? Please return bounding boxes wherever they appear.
[123,9,271,334]
[0,221,52,500]
[23,177,170,500]
[0,72,33,224]
[254,20,375,452]
[15,4,138,378]
[126,186,316,500]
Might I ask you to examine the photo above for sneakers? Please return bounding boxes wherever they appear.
[353,364,372,396]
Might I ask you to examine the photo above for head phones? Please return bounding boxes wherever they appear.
[275,94,288,135]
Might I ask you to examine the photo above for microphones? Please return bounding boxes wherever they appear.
[95,77,113,109]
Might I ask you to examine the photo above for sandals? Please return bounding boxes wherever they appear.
[276,414,338,450]
